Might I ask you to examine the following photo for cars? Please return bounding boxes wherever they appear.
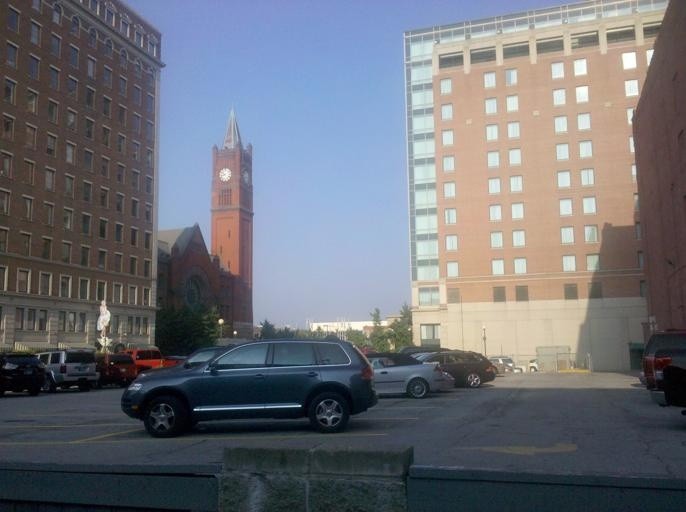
[360,344,539,398]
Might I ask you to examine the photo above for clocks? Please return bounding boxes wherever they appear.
[219,168,232,182]
[242,170,249,185]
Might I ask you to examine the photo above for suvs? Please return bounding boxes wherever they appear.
[0,343,228,397]
[640,327,686,416]
[121,339,378,436]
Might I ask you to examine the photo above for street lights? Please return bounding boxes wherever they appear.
[218,319,224,338]
[233,330,237,338]
[482,325,488,356]
